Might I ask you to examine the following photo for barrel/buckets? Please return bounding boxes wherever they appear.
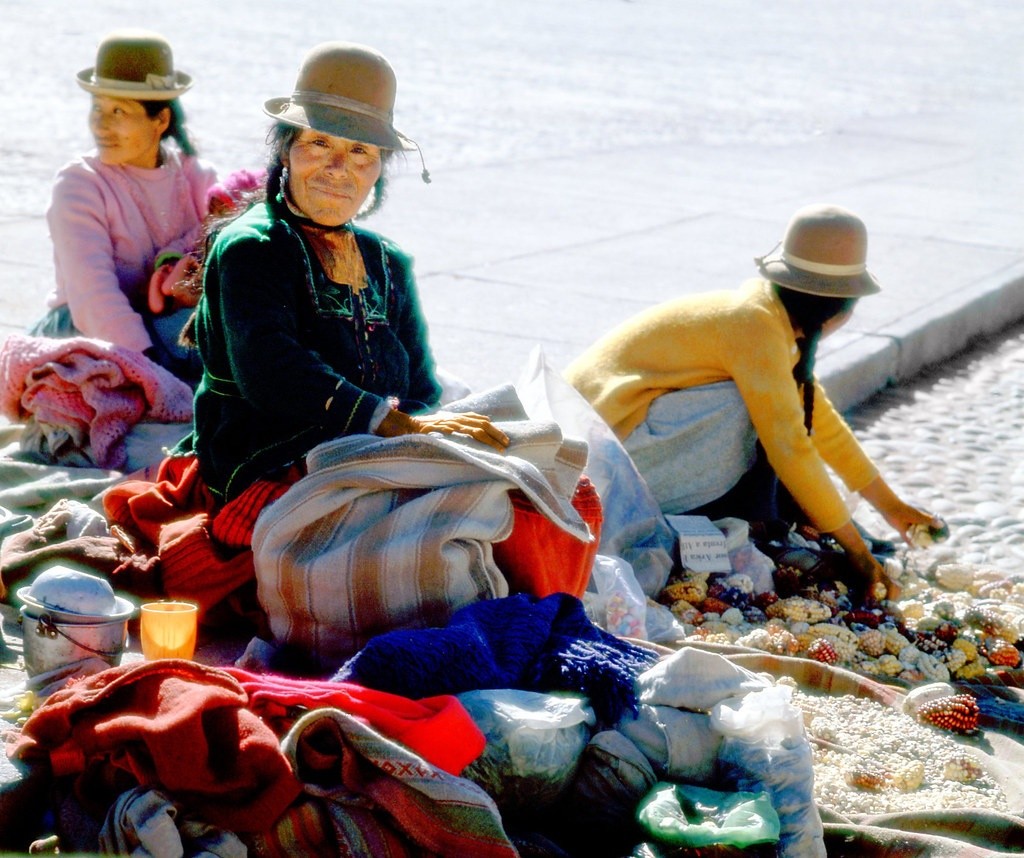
[20,604,129,681]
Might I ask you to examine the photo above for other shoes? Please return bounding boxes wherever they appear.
[817,528,897,558]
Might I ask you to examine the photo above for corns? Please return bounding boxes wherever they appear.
[657,582,1021,791]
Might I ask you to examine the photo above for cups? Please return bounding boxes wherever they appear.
[140,602,199,663]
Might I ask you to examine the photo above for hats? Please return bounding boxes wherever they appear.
[74,35,195,102]
[261,40,418,154]
[755,206,883,300]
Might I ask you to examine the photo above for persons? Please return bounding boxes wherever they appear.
[572,208,946,614]
[185,48,605,662]
[30,36,233,382]
